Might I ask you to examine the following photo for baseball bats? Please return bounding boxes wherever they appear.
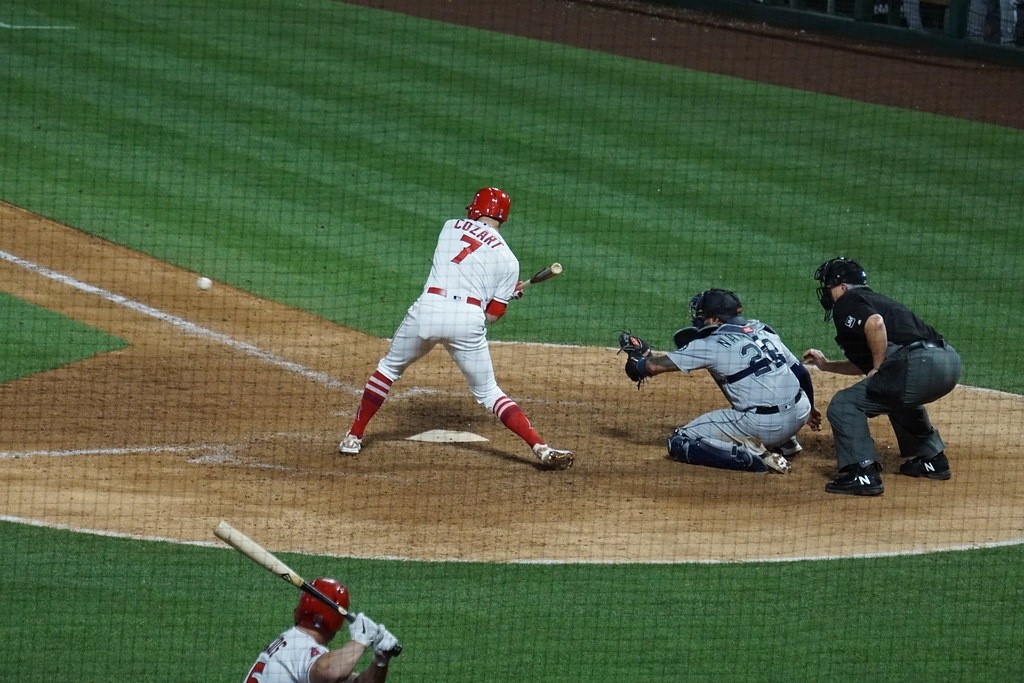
[213,519,404,658]
[516,262,563,290]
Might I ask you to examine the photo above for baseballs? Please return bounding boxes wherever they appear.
[199,277,212,289]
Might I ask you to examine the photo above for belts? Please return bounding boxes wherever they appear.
[905,336,946,351]
[427,287,481,307]
[755,390,802,414]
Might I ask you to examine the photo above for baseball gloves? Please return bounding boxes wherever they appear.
[608,327,656,391]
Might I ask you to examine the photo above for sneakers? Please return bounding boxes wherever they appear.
[533,443,575,469]
[900,450,951,479]
[778,435,802,456]
[825,462,885,495]
[738,435,792,474]
[339,430,362,454]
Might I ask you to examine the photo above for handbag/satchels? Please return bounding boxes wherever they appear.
[865,358,908,405]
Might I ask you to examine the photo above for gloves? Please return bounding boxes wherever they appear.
[349,611,379,648]
[371,623,398,667]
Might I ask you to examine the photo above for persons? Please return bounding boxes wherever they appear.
[625,289,822,473]
[244,578,398,683]
[800,257,963,496]
[338,186,574,471]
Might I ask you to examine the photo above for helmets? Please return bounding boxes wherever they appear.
[294,577,350,641]
[465,187,511,223]
[825,256,867,288]
[700,289,746,325]
